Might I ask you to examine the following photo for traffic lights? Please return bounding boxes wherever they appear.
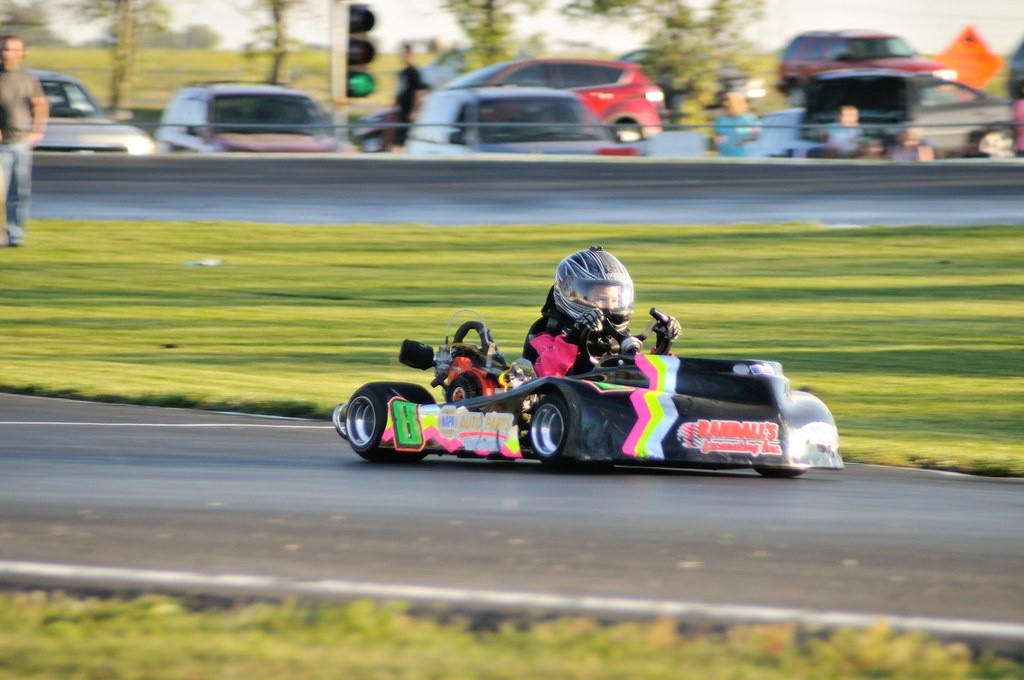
[328,0,378,103]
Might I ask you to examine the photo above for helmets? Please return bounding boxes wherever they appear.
[553,245,634,331]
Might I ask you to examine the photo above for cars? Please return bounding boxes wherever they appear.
[22,68,156,159]
[346,56,667,153]
[404,84,646,161]
[757,29,1023,159]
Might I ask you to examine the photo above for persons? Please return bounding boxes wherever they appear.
[0,34,47,250]
[388,44,425,152]
[675,82,1024,160]
[519,245,682,378]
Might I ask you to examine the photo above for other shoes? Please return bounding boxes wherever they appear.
[9,236,25,247]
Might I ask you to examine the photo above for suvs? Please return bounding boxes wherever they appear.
[152,78,355,153]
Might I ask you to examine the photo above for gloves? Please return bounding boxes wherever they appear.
[652,315,681,351]
[562,308,604,339]
